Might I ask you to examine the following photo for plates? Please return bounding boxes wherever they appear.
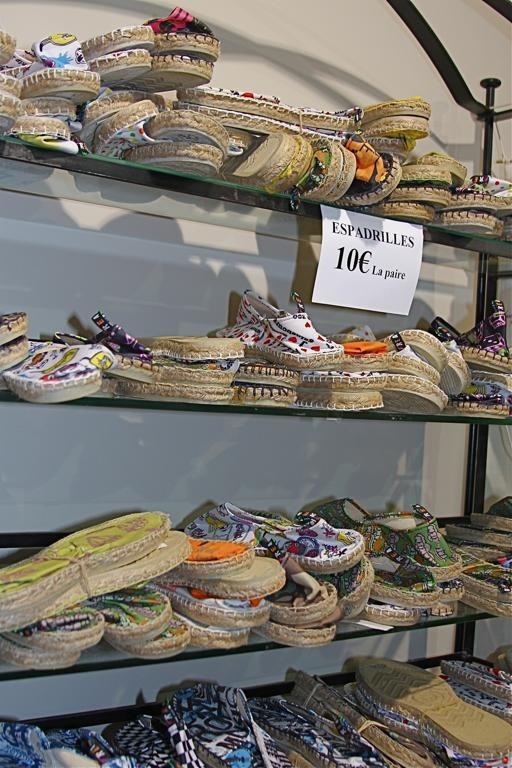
[0,105,512,734]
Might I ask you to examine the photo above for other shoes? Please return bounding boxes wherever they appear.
[0,496,512,671]
[0,8,512,242]
[0,290,511,419]
[0,658,512,768]
